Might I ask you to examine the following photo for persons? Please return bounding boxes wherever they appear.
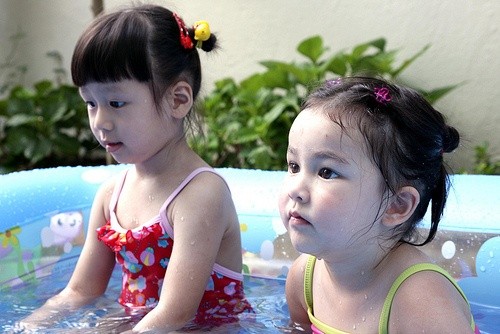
[277,77,482,334]
[6,4,256,334]
